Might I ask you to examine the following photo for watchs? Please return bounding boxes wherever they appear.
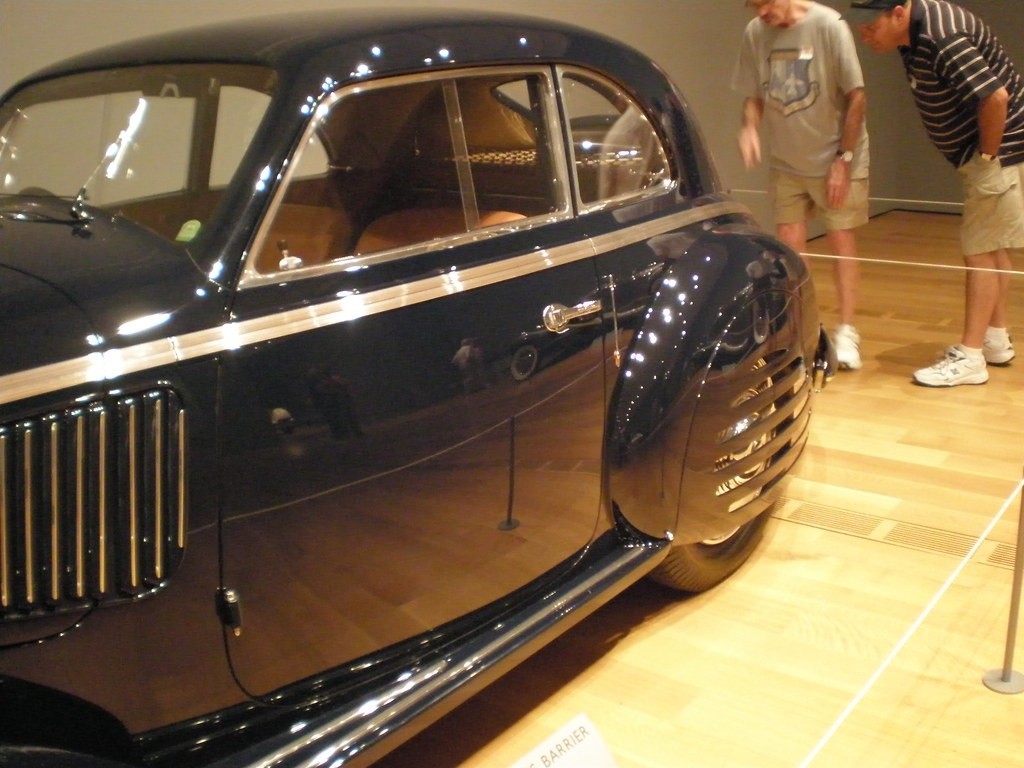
[836,150,854,162]
[979,151,995,161]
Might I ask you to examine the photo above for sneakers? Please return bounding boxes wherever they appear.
[833,324,864,371]
[982,334,1017,364]
[914,345,991,387]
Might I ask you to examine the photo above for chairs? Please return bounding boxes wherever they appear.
[252,204,528,277]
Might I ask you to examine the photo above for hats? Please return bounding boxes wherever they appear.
[839,0,911,23]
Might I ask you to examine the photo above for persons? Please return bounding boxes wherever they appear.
[732,0,869,371]
[840,0,1024,387]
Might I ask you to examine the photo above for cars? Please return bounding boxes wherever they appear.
[0,0,839,768]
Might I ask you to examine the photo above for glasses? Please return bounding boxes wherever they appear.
[744,0,776,8]
